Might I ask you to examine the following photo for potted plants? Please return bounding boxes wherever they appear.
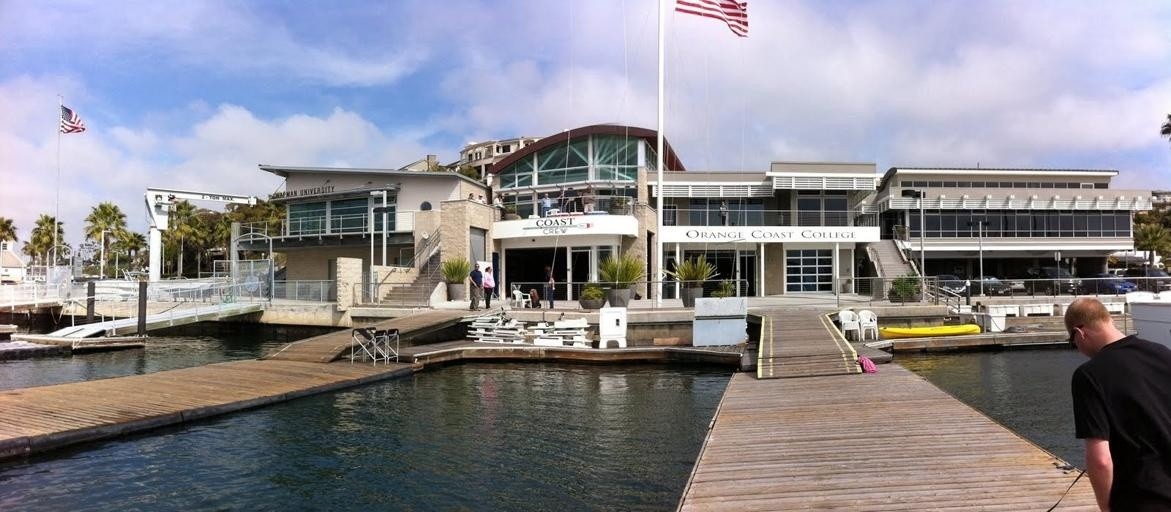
[662,253,722,308]
[504,204,517,220]
[887,271,922,302]
[577,253,649,310]
[610,197,630,215]
[439,257,473,302]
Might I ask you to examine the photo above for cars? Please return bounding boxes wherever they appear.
[935,266,1171,296]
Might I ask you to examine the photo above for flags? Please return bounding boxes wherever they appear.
[664,0,748,37]
[59,105,86,133]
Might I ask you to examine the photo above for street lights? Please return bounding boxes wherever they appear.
[967,220,991,297]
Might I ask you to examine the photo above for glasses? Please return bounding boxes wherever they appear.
[1066,324,1083,350]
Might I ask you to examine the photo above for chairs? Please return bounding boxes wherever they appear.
[512,290,533,309]
[838,310,878,341]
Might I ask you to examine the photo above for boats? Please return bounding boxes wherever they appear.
[879,324,980,338]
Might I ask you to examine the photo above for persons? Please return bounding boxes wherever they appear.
[493,195,506,219]
[469,263,482,310]
[476,194,485,205]
[541,192,551,218]
[24,308,35,332]
[483,267,496,309]
[525,289,541,308]
[468,192,506,220]
[539,188,596,217]
[468,193,473,200]
[1064,298,1171,512]
[719,202,728,225]
[720,202,728,226]
[545,267,556,309]
